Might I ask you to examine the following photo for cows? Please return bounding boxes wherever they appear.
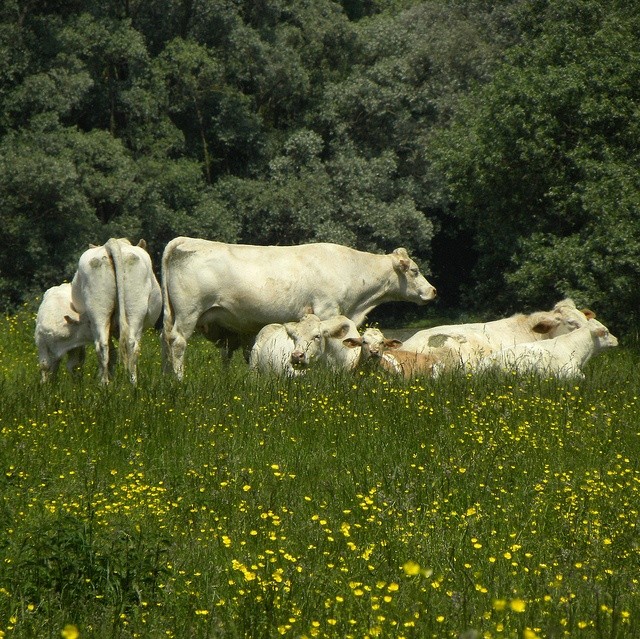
[161,235,438,381]
[395,296,597,386]
[248,313,362,379]
[342,329,446,381]
[34,281,95,382]
[71,236,163,389]
[471,317,620,380]
